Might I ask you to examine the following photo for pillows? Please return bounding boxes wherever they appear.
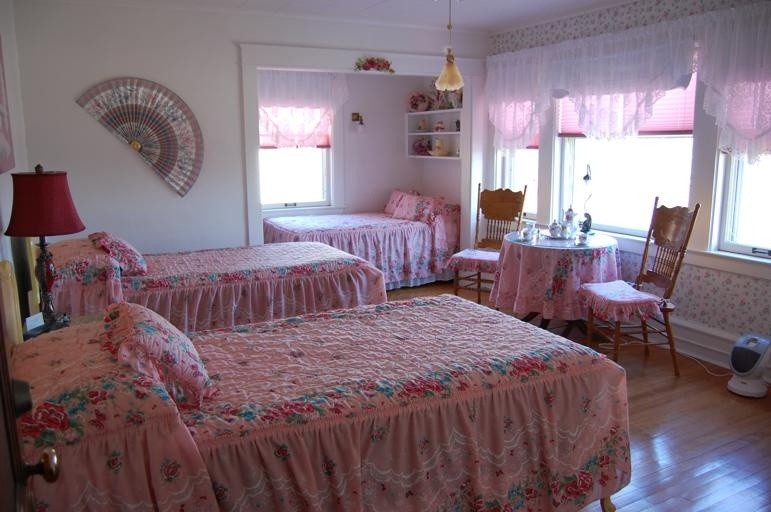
[385,188,461,224]
[88,232,147,275]
[104,298,219,406]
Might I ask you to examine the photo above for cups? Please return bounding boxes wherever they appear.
[523,231,531,240]
[580,233,589,244]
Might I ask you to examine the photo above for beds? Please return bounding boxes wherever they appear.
[45,237,388,333]
[7,291,630,512]
[264,192,462,291]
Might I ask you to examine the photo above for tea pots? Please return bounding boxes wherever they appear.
[547,219,563,237]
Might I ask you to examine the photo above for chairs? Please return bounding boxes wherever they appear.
[450,181,530,310]
[578,196,703,377]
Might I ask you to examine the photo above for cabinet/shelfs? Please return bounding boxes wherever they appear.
[404,107,460,161]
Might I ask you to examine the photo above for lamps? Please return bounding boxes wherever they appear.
[434,1,467,94]
[4,162,88,332]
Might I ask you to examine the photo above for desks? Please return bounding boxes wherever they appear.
[503,229,619,338]
